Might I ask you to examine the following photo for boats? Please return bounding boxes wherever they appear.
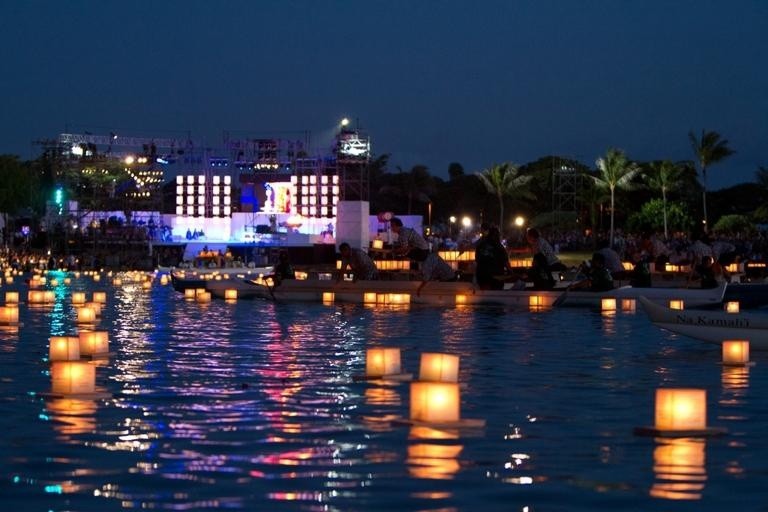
[167,267,728,308]
[638,294,767,352]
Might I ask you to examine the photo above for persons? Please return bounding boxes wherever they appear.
[263,216,768,288]
[0,215,216,272]
